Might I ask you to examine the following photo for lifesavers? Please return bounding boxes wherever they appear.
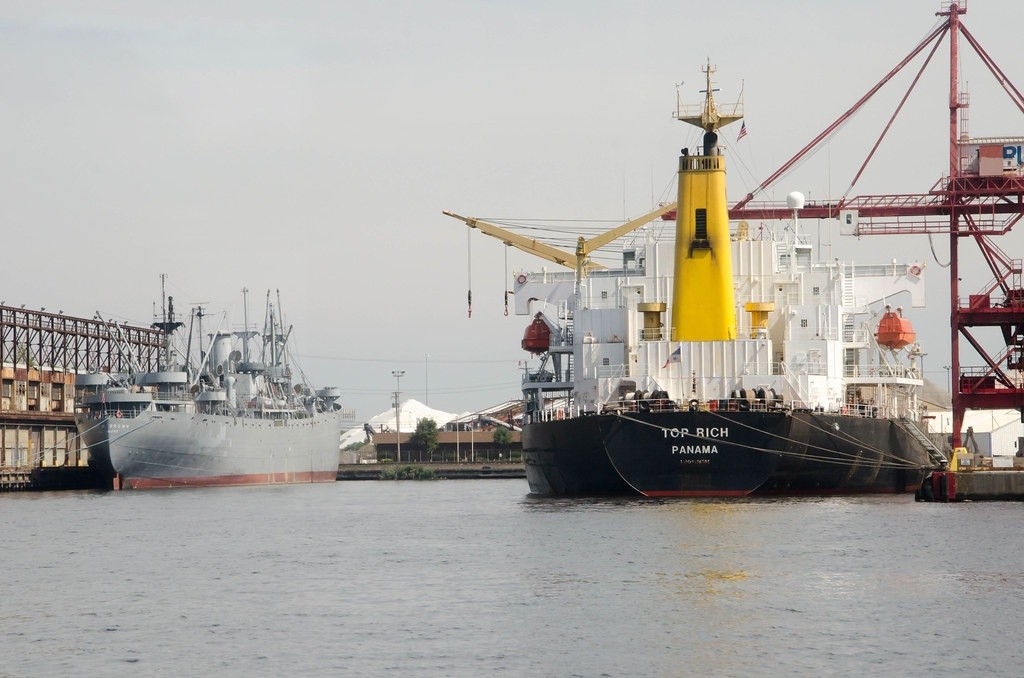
[558,410,563,419]
[841,406,848,416]
[116,411,122,418]
[518,275,526,284]
[910,266,921,275]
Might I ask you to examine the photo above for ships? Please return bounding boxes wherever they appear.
[71,271,343,494]
[443,0,1024,503]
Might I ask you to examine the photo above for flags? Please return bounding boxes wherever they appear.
[736,119,748,141]
